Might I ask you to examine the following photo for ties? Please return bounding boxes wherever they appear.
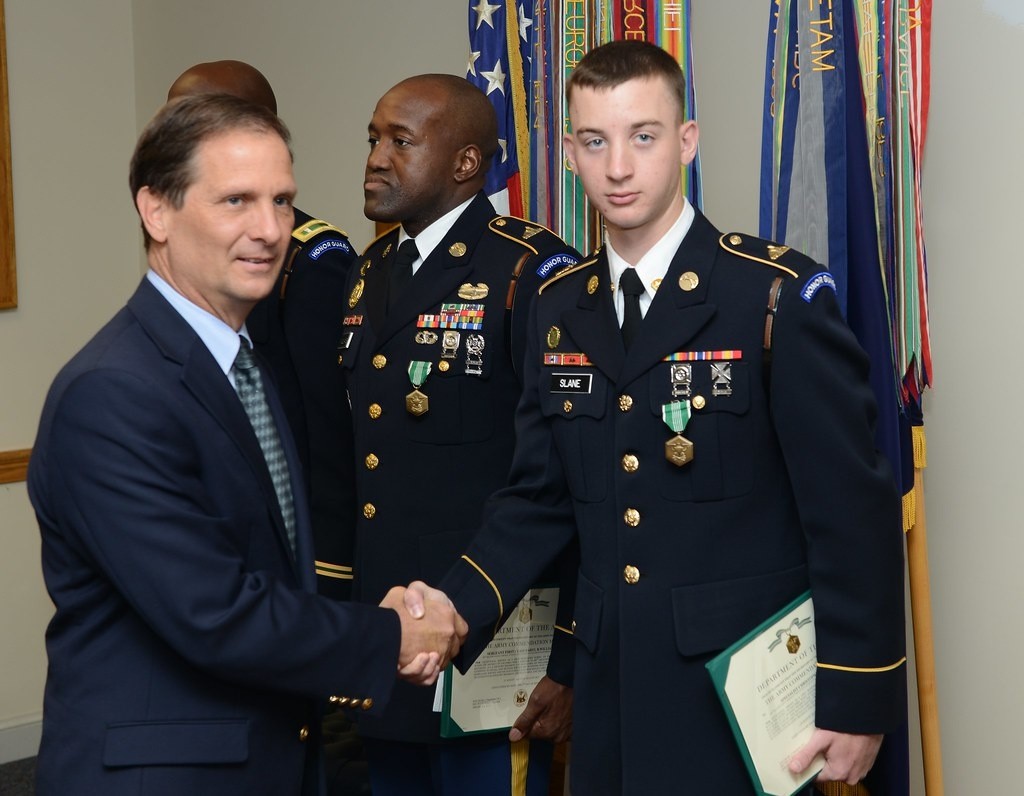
[389,239,419,301]
[619,268,645,353]
[233,335,297,557]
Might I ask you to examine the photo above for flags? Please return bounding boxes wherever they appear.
[466,0,555,233]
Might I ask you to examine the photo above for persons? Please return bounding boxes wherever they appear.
[306,74,585,796]
[26,92,468,796]
[167,60,359,467]
[397,40,906,796]
